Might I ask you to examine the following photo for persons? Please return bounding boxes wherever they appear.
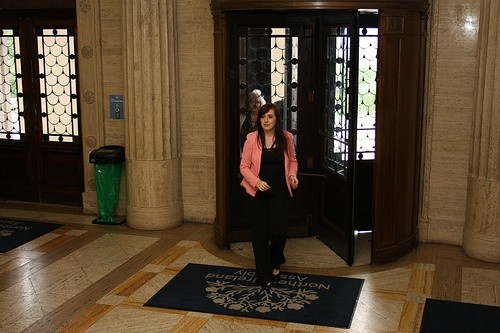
[239,103,299,289]
[238,89,267,179]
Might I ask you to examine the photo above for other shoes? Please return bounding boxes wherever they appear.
[261,276,270,289]
[271,267,282,282]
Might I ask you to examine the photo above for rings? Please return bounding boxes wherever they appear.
[262,188,263,189]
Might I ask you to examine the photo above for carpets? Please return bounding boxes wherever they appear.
[419,298,500,333]
[143,262,365,329]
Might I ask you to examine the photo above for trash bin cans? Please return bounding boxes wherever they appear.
[89,145,125,225]
